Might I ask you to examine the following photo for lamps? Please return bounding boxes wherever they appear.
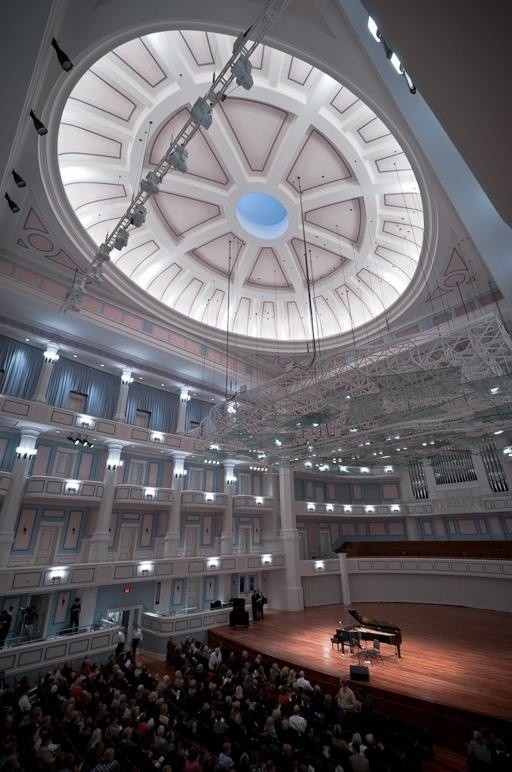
[52,39,74,73]
[139,176,160,196]
[84,266,98,283]
[131,204,148,228]
[30,109,48,137]
[6,193,21,214]
[113,229,129,251]
[93,244,112,261]
[73,281,88,297]
[62,297,81,312]
[12,170,24,195]
[167,148,190,174]
[234,53,255,92]
[191,98,214,130]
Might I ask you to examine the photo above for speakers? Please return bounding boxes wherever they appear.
[349,664,371,682]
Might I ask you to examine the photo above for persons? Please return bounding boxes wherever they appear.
[467,731,512,772]
[0,589,435,772]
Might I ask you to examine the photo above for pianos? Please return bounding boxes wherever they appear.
[336,608,402,659]
